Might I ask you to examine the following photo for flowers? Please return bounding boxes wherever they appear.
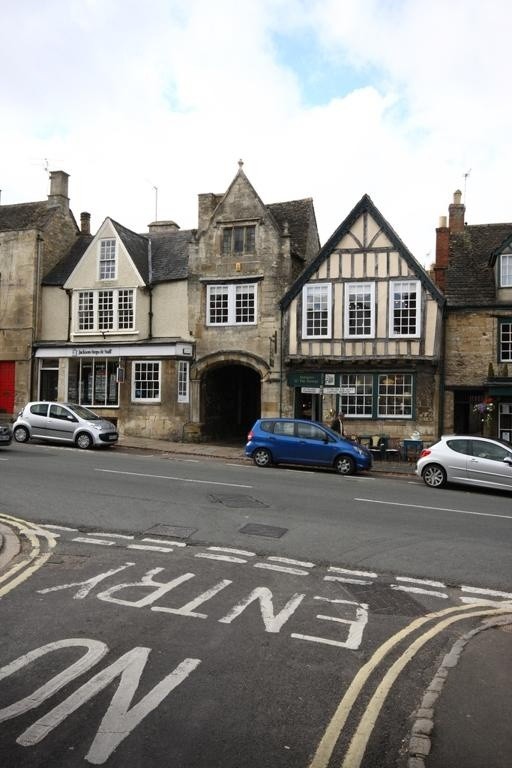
[471,395,496,428]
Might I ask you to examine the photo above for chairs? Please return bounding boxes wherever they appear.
[353,435,401,462]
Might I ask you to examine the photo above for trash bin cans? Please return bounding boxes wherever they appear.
[404,440,423,463]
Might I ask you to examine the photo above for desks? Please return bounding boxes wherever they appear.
[402,438,422,462]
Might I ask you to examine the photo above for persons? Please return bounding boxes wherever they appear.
[331,413,345,437]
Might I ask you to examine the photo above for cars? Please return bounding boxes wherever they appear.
[415,434,512,492]
[0,408,12,446]
[246,418,373,475]
[11,399,119,450]
